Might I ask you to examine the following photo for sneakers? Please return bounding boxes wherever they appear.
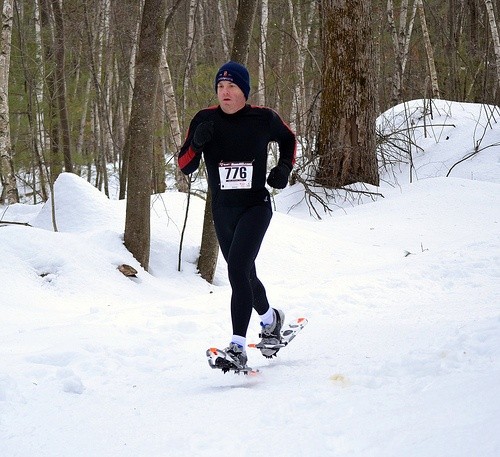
[205,341,248,370]
[258,307,285,359]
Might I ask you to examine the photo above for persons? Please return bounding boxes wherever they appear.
[178,61,297,371]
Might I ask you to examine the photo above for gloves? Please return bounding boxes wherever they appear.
[267,164,289,189]
[190,123,212,153]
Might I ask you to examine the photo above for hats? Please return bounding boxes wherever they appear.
[214,64,251,101]
